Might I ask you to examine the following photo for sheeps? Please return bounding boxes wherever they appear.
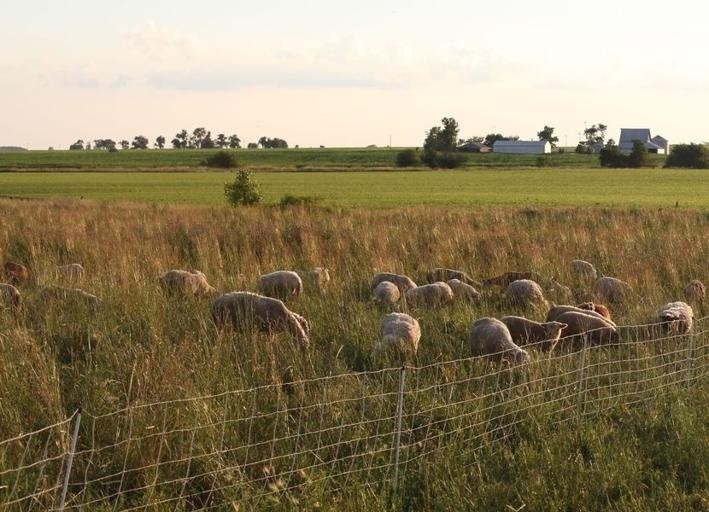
[0,261,28,318]
[40,285,101,308]
[256,268,303,302]
[51,262,86,286]
[370,259,633,370]
[158,268,216,305]
[659,300,695,342]
[682,279,706,318]
[312,266,329,299]
[209,290,310,352]
